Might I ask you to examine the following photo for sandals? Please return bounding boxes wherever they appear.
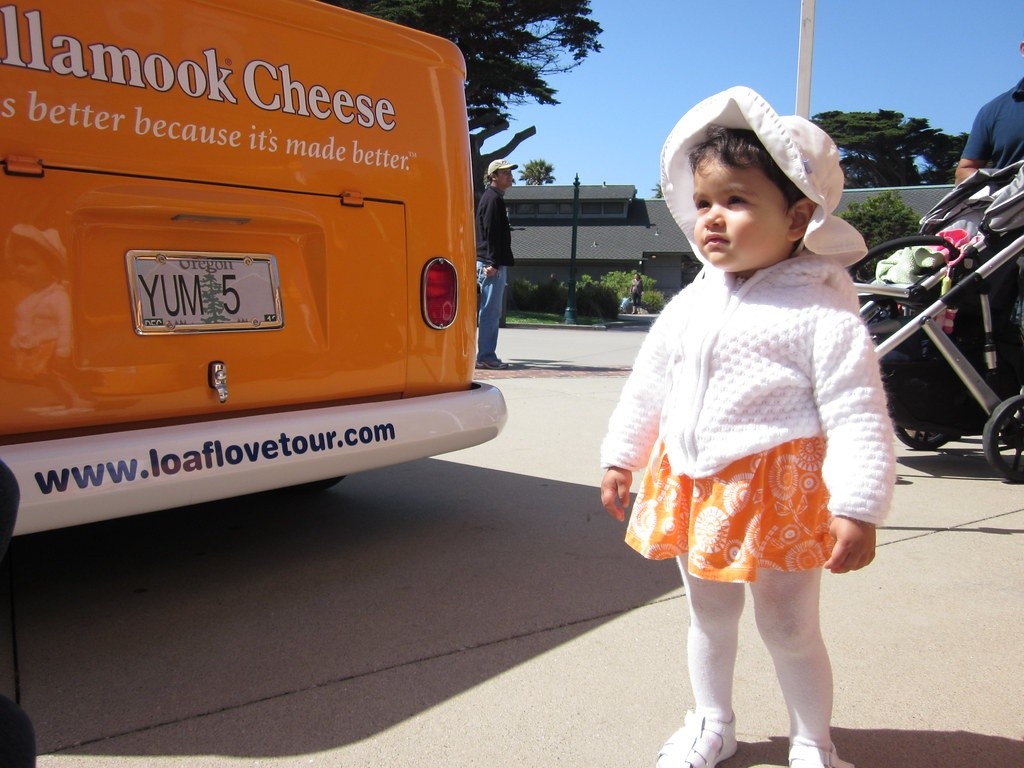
[787,744,857,768]
[654,709,739,768]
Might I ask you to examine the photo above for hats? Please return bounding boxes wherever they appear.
[660,86,868,269]
[488,159,519,175]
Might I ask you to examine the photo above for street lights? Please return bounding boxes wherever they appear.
[564,173,582,327]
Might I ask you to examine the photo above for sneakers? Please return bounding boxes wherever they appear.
[476,360,509,370]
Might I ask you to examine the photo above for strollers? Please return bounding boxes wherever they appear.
[850,161,1024,488]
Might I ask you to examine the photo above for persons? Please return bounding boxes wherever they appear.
[954,38,1024,401]
[630,273,645,314]
[598,83,898,768]
[475,158,519,370]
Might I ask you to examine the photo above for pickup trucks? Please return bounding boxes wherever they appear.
[0,5,508,554]
